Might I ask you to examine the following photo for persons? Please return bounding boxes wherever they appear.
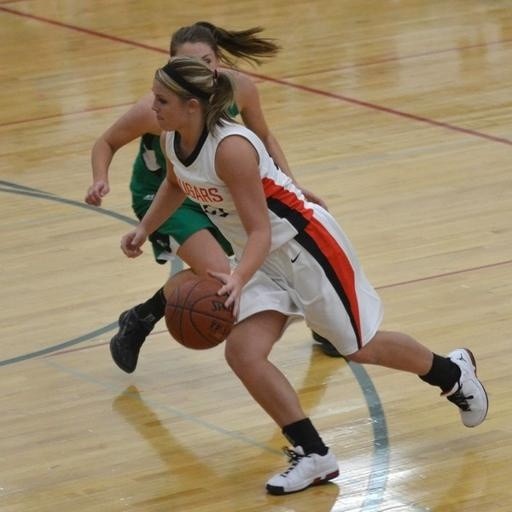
[83,22,354,377]
[115,57,488,496]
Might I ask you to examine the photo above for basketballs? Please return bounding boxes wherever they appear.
[165,274,235,349]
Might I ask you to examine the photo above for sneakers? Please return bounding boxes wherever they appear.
[438,345,491,430]
[108,302,159,375]
[263,444,341,495]
